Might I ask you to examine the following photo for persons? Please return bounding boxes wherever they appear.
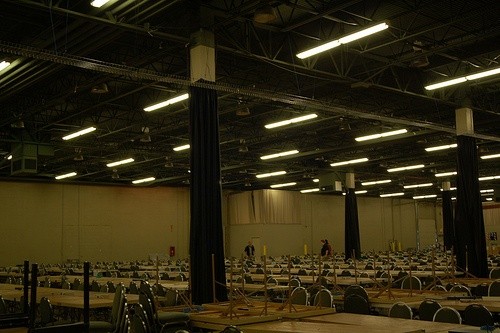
[243,240,257,258]
[319,239,329,257]
[324,237,331,255]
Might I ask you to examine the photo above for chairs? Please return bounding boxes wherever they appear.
[0,261,500,333]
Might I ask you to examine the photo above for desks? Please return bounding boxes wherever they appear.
[190,241,500,333]
[39,275,189,290]
[0,284,169,322]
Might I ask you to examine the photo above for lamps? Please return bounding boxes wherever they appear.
[0,0,500,201]
